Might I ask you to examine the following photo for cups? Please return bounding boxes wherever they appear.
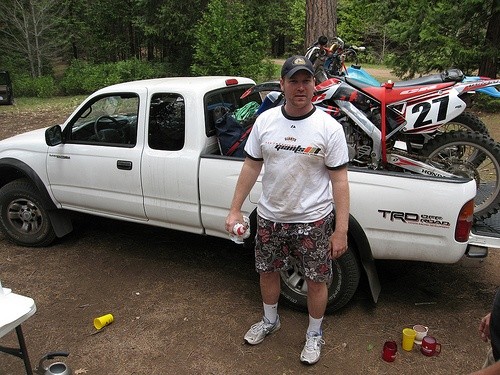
[95,314,113,330]
[413,325,429,345]
[421,336,442,357]
[403,329,416,352]
[382,341,399,362]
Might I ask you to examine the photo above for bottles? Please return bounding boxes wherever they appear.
[232,221,248,236]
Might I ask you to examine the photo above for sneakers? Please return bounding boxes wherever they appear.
[300,328,325,364]
[243,314,281,344]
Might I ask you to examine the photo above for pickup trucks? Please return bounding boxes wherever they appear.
[1,76,500,315]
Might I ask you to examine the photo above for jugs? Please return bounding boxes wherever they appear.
[34,351,71,375]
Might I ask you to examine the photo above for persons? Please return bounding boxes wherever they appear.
[470,285,500,375]
[225,56,349,365]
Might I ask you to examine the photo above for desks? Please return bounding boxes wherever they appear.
[0,288,37,375]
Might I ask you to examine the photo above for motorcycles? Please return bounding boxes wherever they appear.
[215,37,500,224]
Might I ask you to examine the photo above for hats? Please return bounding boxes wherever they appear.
[281,56,315,79]
